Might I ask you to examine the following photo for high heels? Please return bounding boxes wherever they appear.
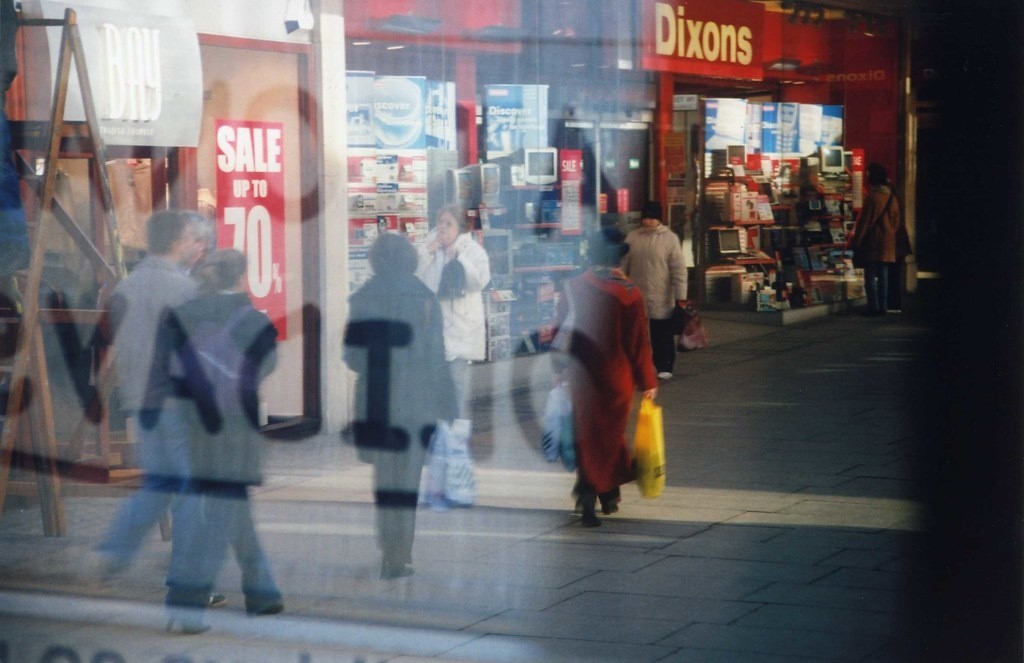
[246,600,283,615]
[167,615,212,634]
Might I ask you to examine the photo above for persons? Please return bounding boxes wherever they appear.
[341,233,445,580]
[128,248,282,635]
[616,197,690,382]
[411,206,491,463]
[77,209,229,590]
[553,228,656,529]
[852,163,899,318]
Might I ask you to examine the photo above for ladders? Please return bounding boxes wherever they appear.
[1,9,176,542]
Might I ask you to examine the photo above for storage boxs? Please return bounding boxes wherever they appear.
[483,290,512,316]
[539,200,563,224]
[487,313,511,337]
[488,336,512,362]
[513,280,557,355]
[347,147,429,294]
[444,161,471,209]
[464,163,501,208]
[704,145,867,313]
[513,241,575,267]
[481,225,512,279]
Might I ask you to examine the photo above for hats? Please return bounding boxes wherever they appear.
[868,169,890,185]
[641,200,663,223]
[373,233,420,273]
[587,229,631,264]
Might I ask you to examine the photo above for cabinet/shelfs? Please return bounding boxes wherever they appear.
[802,193,851,249]
[512,182,577,273]
[735,218,777,266]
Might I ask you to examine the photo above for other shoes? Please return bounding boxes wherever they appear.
[165,590,228,608]
[81,554,138,599]
[574,488,619,527]
[860,304,887,315]
[380,560,413,580]
[658,371,673,379]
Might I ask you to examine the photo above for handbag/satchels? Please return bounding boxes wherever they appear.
[635,394,665,498]
[544,381,576,475]
[677,300,708,352]
[852,237,873,269]
[436,260,466,299]
[425,418,476,512]
